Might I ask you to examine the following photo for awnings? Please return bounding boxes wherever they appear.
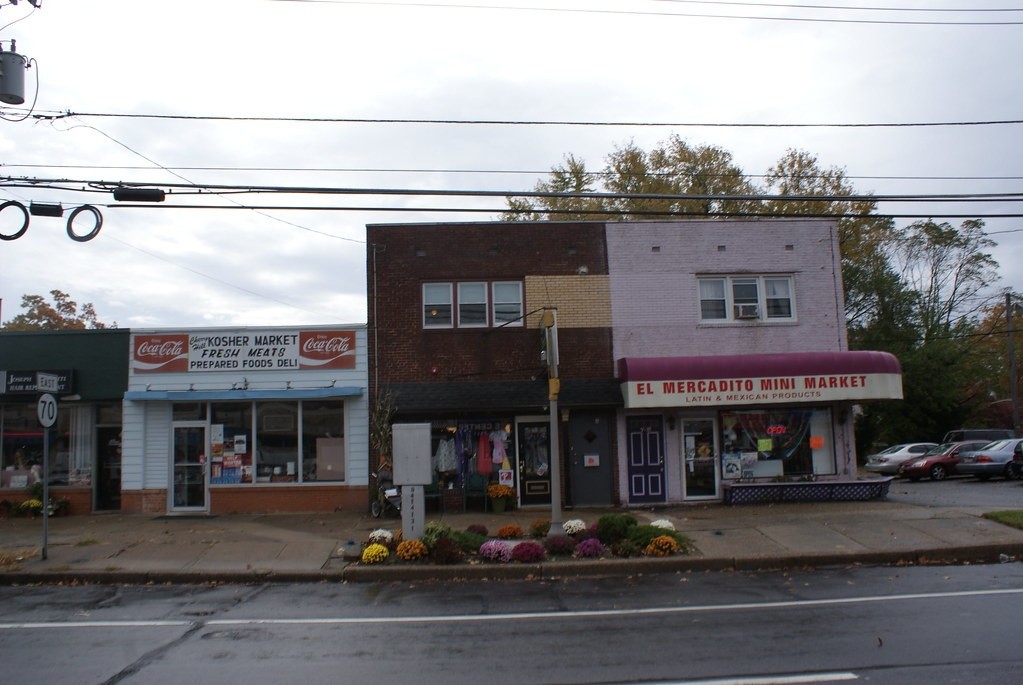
[617,350,904,408]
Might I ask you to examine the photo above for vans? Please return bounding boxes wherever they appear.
[939,429,1015,452]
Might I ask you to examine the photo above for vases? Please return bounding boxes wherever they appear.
[491,497,505,513]
[27,512,32,516]
[59,509,64,517]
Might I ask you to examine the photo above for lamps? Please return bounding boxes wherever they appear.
[146,382,151,390]
[286,380,291,388]
[332,379,336,386]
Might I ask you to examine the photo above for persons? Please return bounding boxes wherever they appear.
[14,449,24,465]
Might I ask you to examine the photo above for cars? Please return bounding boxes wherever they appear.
[955,437,1023,481]
[1006,439,1023,481]
[896,439,994,482]
[862,443,939,476]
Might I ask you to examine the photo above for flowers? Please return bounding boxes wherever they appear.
[488,485,512,498]
[16,480,69,515]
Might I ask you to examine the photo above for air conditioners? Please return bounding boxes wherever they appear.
[734,303,760,318]
